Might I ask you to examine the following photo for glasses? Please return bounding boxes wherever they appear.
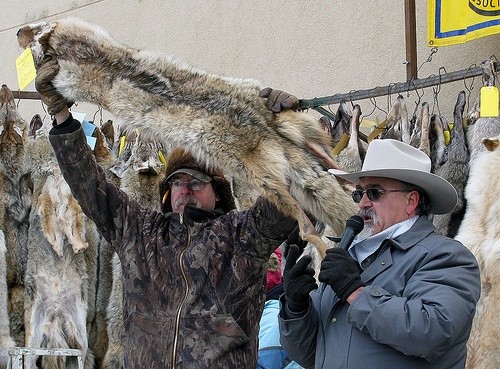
[352,188,413,202]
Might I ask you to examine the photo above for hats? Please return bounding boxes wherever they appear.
[328,138,458,214]
[169,179,205,190]
[163,147,224,182]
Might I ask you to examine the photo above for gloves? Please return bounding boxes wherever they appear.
[283,244,318,310]
[34,55,77,114]
[258,88,299,112]
[319,247,365,302]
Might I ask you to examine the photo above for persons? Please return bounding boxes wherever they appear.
[277,138,482,368]
[36,61,301,369]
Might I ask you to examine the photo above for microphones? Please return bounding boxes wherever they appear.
[322,216,364,291]
